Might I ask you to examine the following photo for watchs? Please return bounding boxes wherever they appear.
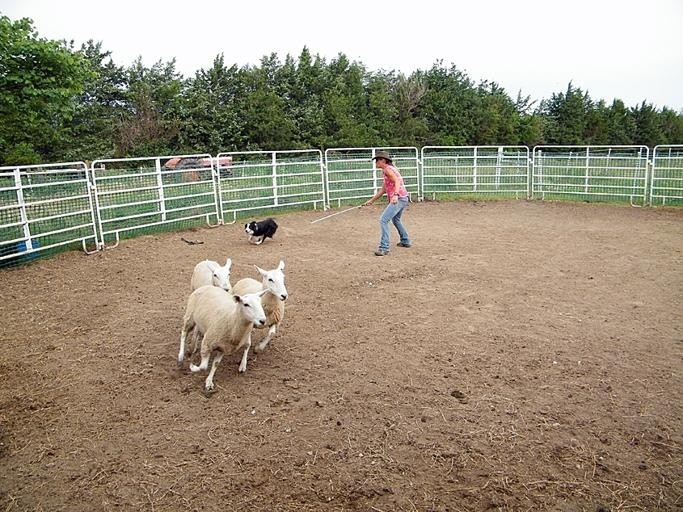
[393,192,399,196]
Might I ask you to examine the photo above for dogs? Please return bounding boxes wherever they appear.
[244,217,279,244]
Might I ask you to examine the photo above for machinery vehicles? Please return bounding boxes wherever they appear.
[160,156,232,182]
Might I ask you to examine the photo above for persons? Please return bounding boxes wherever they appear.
[365,151,412,256]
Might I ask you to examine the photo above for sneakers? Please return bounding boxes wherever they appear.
[374,249,390,256]
[396,242,410,247]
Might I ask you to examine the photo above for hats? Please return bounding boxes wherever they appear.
[371,151,392,162]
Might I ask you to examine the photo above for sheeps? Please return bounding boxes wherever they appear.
[188,256,233,291]
[177,284,270,392]
[235,259,289,373]
